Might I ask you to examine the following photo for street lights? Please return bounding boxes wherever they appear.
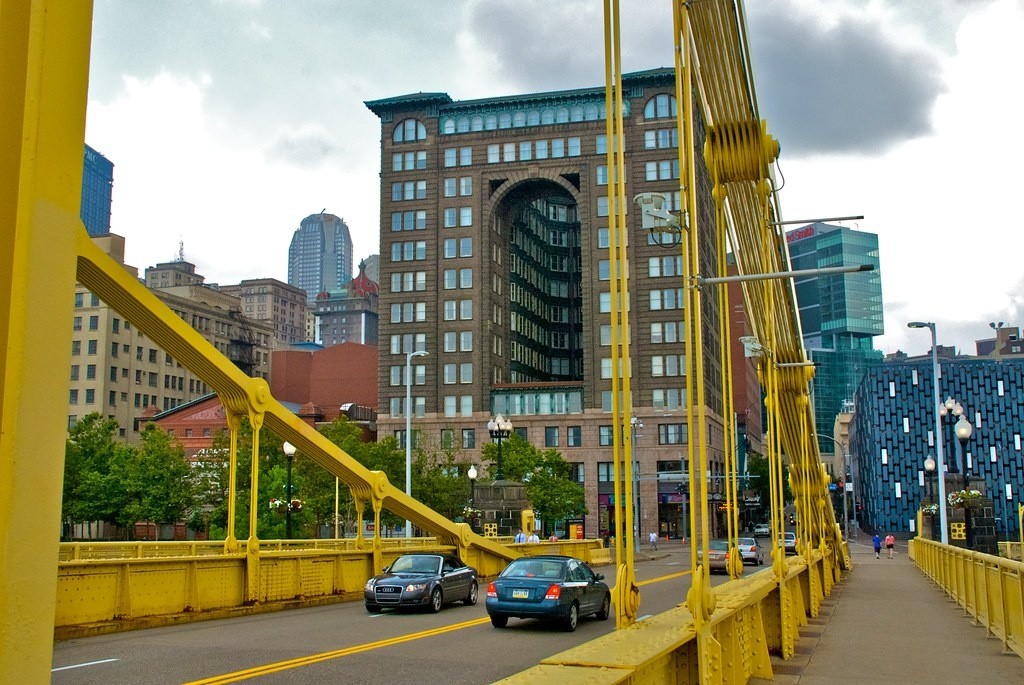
[468,465,478,533]
[923,454,936,540]
[939,395,964,473]
[487,412,512,480]
[629,416,643,553]
[810,432,850,542]
[282,440,297,538]
[405,351,430,539]
[954,414,975,550]
[907,321,949,546]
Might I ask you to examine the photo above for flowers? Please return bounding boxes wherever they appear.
[922,503,939,515]
[946,487,982,506]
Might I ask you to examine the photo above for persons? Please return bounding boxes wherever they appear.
[872,531,882,559]
[527,530,539,543]
[515,528,526,542]
[548,530,558,542]
[648,530,658,552]
[885,532,895,559]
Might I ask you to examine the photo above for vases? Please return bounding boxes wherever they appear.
[963,497,985,508]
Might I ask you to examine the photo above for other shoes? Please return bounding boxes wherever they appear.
[891,557,893,559]
[877,556,879,559]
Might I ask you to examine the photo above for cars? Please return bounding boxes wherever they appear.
[789,513,800,526]
[732,537,764,566]
[363,553,478,614]
[778,532,797,555]
[697,539,744,574]
[485,554,611,633]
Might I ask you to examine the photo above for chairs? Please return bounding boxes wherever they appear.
[514,569,525,574]
[544,569,558,575]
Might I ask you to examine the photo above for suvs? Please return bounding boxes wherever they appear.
[753,524,770,538]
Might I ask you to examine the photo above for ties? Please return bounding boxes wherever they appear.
[519,533,521,543]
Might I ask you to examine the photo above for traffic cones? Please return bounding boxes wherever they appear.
[666,535,669,542]
[682,536,685,544]
